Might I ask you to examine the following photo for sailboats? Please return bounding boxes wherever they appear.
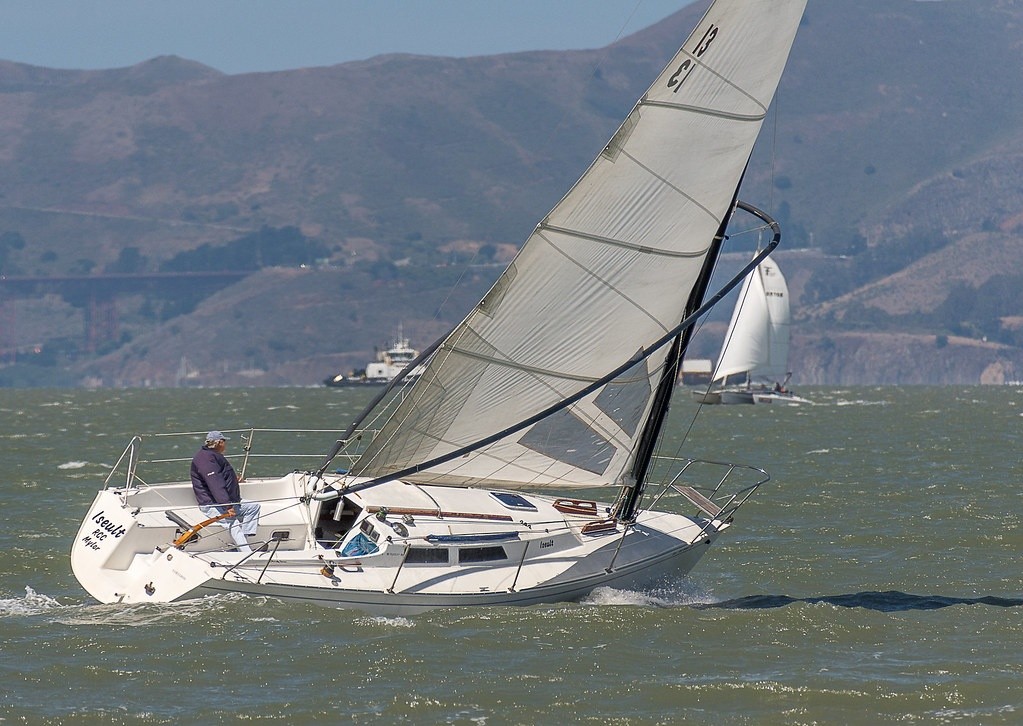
[65,0,812,616]
[690,228,814,408]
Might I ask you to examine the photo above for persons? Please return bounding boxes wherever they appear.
[190,431,262,553]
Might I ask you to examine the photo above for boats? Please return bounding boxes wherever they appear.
[325,324,426,387]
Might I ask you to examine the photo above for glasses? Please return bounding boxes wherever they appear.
[220,439,225,441]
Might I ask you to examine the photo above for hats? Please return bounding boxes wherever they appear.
[206,431,231,441]
